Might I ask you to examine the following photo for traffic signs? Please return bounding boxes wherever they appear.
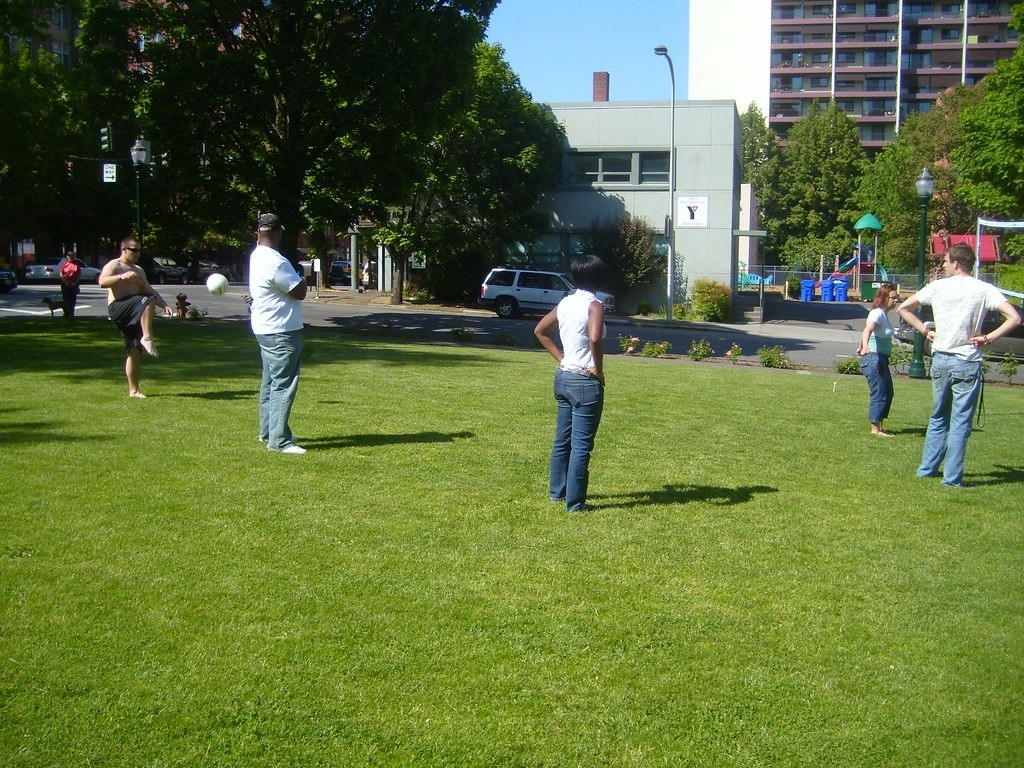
[103,163,118,183]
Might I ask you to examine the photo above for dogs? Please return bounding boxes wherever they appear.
[43,297,64,318]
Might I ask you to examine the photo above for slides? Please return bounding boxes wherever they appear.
[828,257,858,280]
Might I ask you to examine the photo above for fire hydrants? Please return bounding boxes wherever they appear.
[174,292,191,319]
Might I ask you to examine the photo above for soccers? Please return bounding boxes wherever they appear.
[206,272,229,296]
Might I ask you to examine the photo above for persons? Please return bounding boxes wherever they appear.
[858,283,899,438]
[896,242,1022,486]
[99,236,173,399]
[249,213,308,454]
[59,251,81,317]
[534,253,607,513]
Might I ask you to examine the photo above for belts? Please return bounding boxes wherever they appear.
[559,364,594,377]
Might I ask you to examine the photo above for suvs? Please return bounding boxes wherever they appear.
[476,266,616,318]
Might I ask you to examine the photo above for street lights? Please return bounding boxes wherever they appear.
[129,137,146,269]
[654,43,676,323]
[908,163,936,378]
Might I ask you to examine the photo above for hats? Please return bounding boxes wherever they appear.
[258,213,285,231]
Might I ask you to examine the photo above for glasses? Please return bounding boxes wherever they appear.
[126,248,142,253]
[887,294,899,299]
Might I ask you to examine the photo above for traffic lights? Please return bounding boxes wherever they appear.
[100,124,113,154]
[66,161,74,179]
[150,155,158,179]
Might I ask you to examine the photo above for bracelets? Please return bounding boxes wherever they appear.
[983,335,990,345]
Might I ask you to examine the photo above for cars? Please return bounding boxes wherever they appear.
[147,256,191,285]
[893,301,1024,364]
[185,259,231,284]
[25,257,101,285]
[361,262,377,289]
[0,267,19,295]
[328,261,363,287]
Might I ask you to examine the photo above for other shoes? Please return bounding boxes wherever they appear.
[269,445,307,454]
[259,437,299,442]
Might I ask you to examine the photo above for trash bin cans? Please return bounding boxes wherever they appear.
[820,280,834,302]
[861,281,892,302]
[801,279,815,301]
[835,278,847,302]
